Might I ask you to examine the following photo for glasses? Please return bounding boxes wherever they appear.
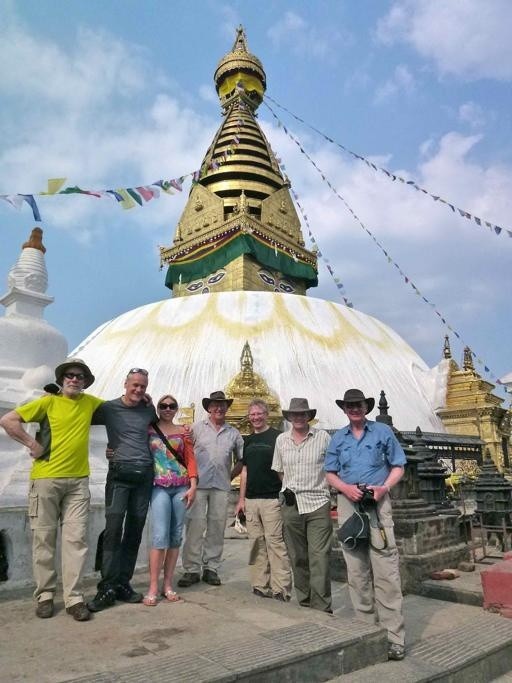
[63,373,86,380]
[127,368,148,378]
[160,403,176,410]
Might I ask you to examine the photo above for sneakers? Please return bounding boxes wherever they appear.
[36,599,53,618]
[66,569,221,620]
[388,644,404,659]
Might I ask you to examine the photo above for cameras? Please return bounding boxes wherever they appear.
[359,488,377,513]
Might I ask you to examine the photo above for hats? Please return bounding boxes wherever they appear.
[203,391,233,413]
[282,389,375,421]
[55,359,94,389]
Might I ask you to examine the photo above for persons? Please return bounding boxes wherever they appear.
[270,397,334,615]
[105,393,199,608]
[323,388,407,659]
[176,391,246,588]
[39,367,195,612]
[233,398,291,601]
[0,358,154,622]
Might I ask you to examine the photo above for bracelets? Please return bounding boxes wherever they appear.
[182,424,186,427]
[384,482,392,493]
[233,471,239,476]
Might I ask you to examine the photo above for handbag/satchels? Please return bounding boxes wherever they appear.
[337,512,369,545]
